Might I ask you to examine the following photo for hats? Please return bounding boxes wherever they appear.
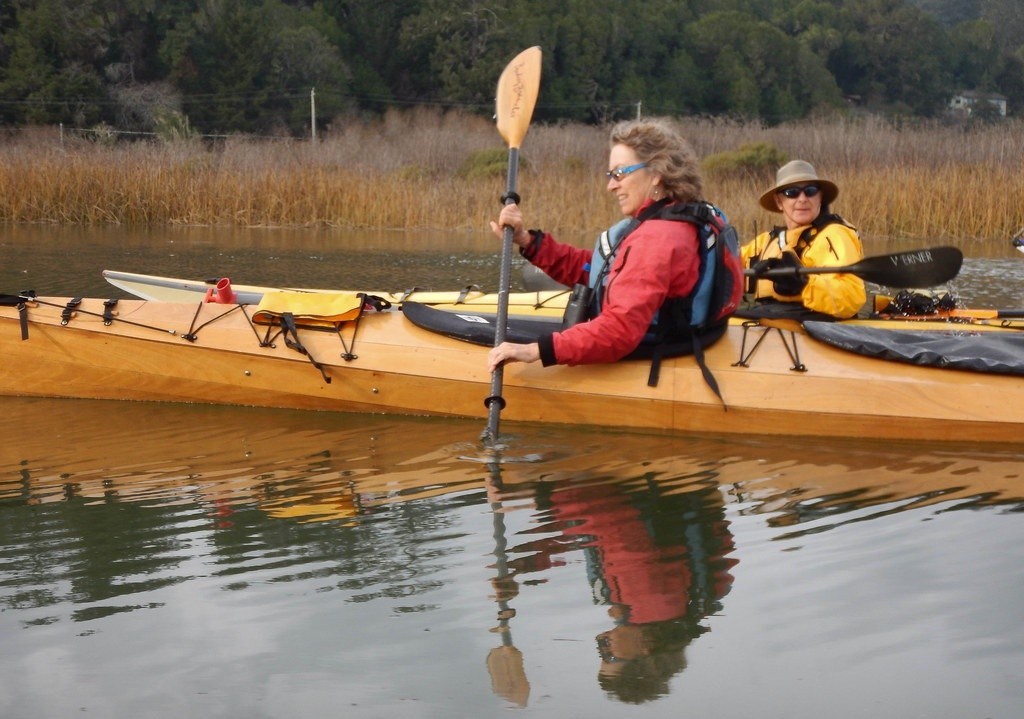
[758,159,839,213]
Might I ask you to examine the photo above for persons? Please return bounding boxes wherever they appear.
[488,119,727,381]
[739,160,867,318]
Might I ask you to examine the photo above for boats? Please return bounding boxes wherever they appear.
[102,270,1024,332]
[1,287,1024,442]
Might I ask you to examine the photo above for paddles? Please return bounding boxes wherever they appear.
[484,45,542,449]
[742,246,962,289]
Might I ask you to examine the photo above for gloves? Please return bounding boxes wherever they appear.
[753,250,809,297]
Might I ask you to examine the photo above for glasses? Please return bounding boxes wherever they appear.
[776,185,822,199]
[605,162,649,182]
[599,652,637,664]
